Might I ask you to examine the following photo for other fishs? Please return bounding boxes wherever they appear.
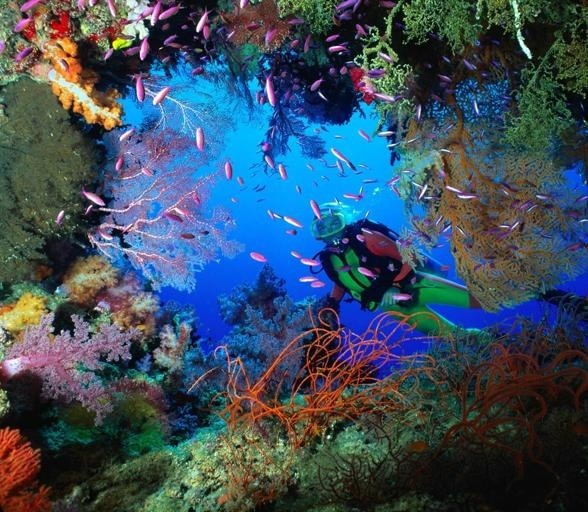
[1,0,588,304]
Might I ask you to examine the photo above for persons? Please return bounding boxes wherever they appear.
[308,200,587,355]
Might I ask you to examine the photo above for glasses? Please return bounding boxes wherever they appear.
[309,212,347,240]
